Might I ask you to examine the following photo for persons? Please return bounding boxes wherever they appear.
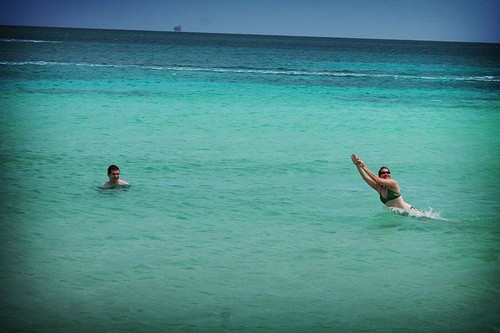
[99,164,130,190]
[350,153,420,214]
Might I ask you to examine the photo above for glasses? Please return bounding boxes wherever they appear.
[378,171,391,174]
